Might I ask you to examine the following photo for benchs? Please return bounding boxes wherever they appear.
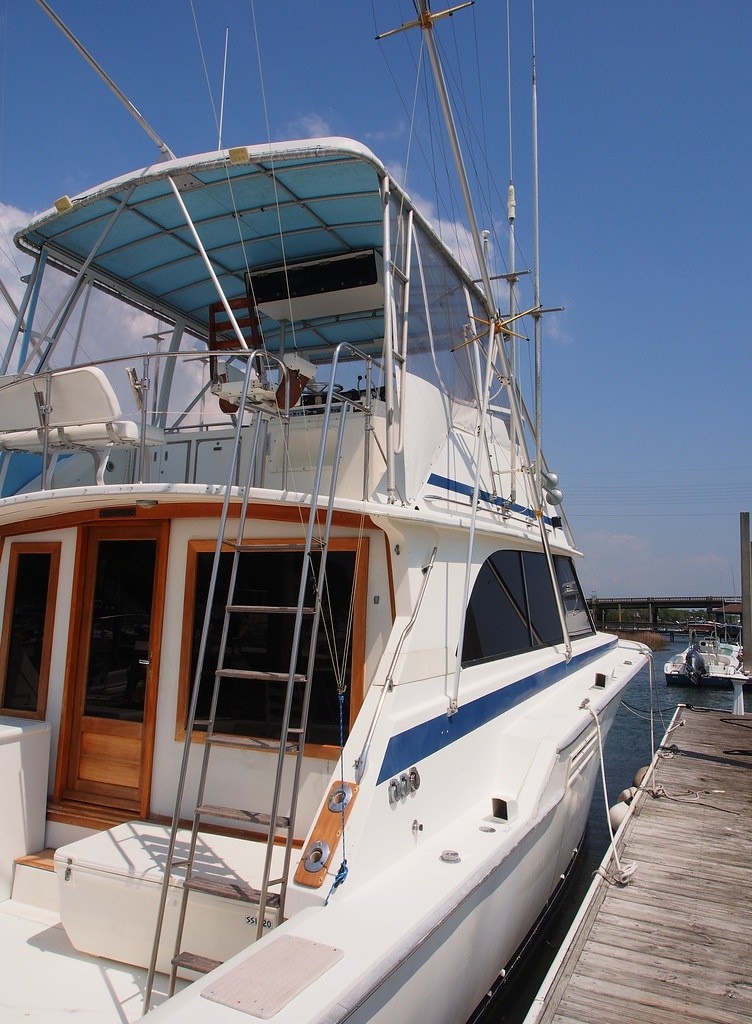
[0,368,165,497]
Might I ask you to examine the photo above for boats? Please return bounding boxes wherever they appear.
[0,0,650,1024]
[663,597,752,689]
